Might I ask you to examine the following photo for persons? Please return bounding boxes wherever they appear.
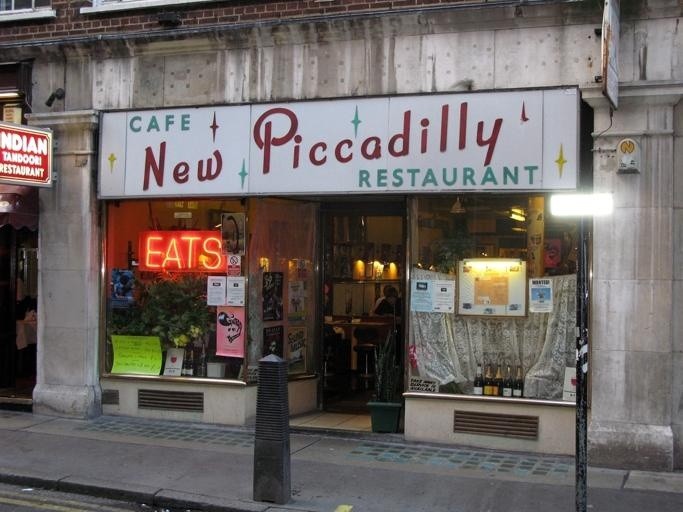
[368,284,392,317]
[351,311,378,392]
[111,275,132,299]
[266,336,279,358]
[261,273,283,321]
[373,285,401,368]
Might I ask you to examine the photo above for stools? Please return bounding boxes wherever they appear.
[353,343,379,390]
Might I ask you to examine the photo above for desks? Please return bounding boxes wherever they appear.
[324,315,402,392]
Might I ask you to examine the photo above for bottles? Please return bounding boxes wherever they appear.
[502,365,513,396]
[182,351,188,376]
[484,364,494,395]
[494,364,503,396]
[474,363,484,394]
[188,350,196,376]
[513,366,523,398]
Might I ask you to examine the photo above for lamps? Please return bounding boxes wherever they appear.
[461,258,522,266]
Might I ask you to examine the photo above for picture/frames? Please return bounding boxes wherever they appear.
[455,260,529,319]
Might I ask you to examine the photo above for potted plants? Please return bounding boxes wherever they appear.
[367,325,405,434]
[206,348,226,378]
